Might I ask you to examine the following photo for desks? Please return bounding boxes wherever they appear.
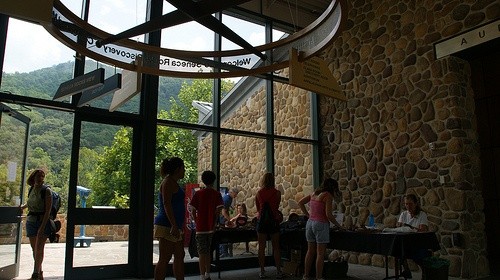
[325,226,442,280]
[189,226,306,279]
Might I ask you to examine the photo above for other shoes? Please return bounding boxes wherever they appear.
[401,270,413,278]
[259,273,265,277]
[303,274,310,280]
[276,270,282,278]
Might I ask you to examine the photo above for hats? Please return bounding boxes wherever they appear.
[49,219,61,233]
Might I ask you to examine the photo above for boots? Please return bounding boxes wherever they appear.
[28,271,44,280]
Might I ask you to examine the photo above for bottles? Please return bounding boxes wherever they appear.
[368,213,374,227]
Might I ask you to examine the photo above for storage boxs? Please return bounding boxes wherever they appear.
[283,248,302,274]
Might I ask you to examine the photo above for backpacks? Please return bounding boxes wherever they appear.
[28,185,61,220]
[258,189,280,234]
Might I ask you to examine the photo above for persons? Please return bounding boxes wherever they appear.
[397,194,428,279]
[189,171,224,280]
[254,173,282,278]
[298,178,344,280]
[19,170,52,280]
[229,203,248,227]
[217,187,239,257]
[154,157,185,280]
